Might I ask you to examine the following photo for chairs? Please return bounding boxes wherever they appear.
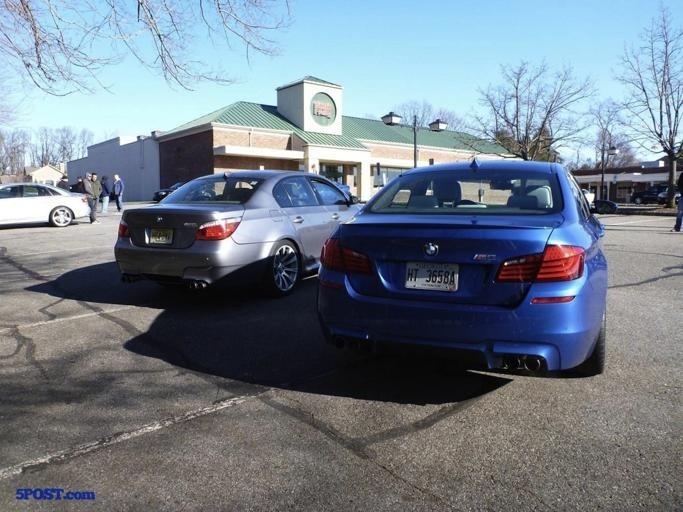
[10,187,47,196]
[276,184,304,208]
[407,182,552,208]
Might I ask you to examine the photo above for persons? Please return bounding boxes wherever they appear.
[667,171,682,232]
[55,174,72,192]
[111,173,124,212]
[99,175,110,217]
[72,176,85,193]
[86,172,102,224]
[82,171,93,202]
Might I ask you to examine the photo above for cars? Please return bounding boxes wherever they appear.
[632,183,681,205]
[0,183,91,227]
[114,157,617,376]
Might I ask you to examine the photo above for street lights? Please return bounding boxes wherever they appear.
[380,111,448,168]
[592,143,620,200]
